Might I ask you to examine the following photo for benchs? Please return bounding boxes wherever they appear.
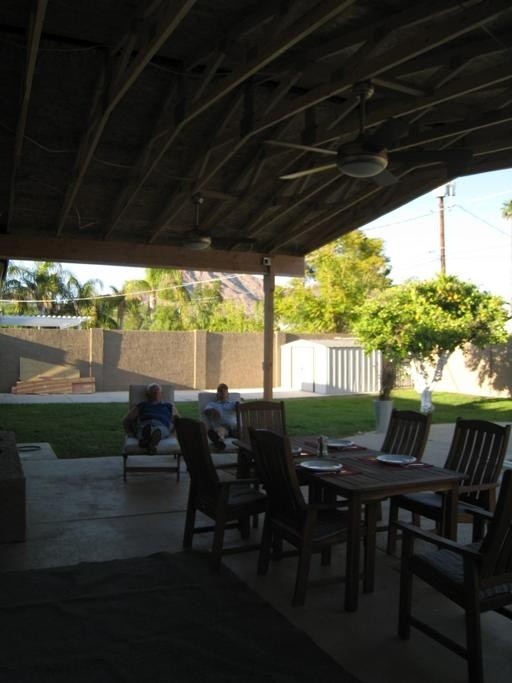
[0,430,27,543]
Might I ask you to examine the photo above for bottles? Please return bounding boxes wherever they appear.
[318,434,329,456]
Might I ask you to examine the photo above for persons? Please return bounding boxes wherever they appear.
[204,383,239,449]
[122,382,180,448]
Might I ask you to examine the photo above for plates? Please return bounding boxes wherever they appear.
[376,454,417,465]
[290,444,302,453]
[300,460,344,471]
[324,438,353,446]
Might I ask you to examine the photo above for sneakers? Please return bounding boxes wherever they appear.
[207,428,227,451]
[138,423,162,456]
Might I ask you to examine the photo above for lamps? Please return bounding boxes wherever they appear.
[182,241,211,251]
[336,154,389,180]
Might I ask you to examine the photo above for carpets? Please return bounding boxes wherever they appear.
[0,548,361,682]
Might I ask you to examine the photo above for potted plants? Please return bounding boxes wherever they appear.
[349,286,437,434]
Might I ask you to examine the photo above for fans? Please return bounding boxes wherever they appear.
[190,193,259,247]
[264,81,455,188]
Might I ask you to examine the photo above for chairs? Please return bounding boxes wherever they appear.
[393,470,512,683]
[230,399,288,527]
[171,414,281,571]
[197,392,244,470]
[121,382,185,484]
[329,408,432,528]
[247,425,377,607]
[385,415,512,556]
